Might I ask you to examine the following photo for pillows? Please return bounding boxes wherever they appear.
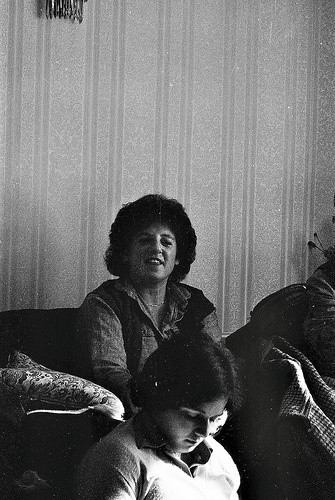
[0,349,125,422]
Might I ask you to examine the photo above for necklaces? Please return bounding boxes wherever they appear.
[146,301,164,306]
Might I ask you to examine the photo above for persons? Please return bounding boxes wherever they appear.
[74,331,241,500]
[72,194,227,418]
[306,259,335,378]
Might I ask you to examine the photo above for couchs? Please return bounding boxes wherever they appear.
[0,283,308,500]
